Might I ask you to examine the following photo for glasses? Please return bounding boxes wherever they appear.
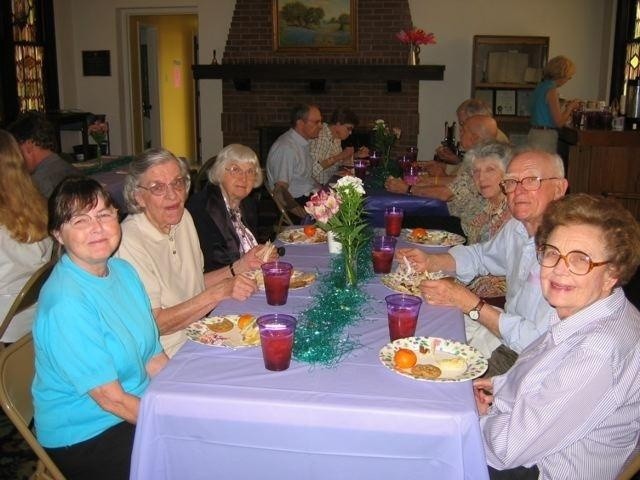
[499,177,559,192]
[226,165,258,180]
[136,178,187,195]
[536,244,612,275]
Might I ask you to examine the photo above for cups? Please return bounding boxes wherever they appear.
[385,295,421,342]
[352,146,418,186]
[256,314,296,371]
[326,230,342,255]
[370,211,404,275]
[262,262,293,306]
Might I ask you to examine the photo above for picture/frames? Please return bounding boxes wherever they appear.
[270,0,359,55]
[473,88,536,118]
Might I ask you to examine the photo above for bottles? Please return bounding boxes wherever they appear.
[561,100,606,129]
[211,49,219,65]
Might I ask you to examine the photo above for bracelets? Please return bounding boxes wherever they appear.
[230,263,235,277]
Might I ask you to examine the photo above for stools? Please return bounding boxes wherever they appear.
[73,144,107,162]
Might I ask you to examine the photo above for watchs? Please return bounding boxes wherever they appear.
[469,299,486,321]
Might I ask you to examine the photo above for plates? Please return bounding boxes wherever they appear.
[381,227,467,292]
[278,226,325,245]
[184,314,262,349]
[236,267,318,290]
[379,335,489,384]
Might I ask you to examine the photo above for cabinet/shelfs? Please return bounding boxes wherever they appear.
[471,34,551,145]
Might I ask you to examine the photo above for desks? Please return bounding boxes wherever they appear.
[58,112,106,161]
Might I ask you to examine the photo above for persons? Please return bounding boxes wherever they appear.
[395,145,569,378]
[264,97,515,298]
[0,129,62,347]
[193,143,276,272]
[31,174,169,480]
[111,147,280,360]
[528,55,580,153]
[6,111,83,199]
[472,192,640,480]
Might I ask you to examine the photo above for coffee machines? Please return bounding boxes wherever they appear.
[613,75,639,131]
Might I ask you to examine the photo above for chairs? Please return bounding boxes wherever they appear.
[261,171,296,235]
[0,257,58,348]
[0,331,69,480]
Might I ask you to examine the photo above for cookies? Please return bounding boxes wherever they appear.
[206,319,233,333]
[411,364,441,379]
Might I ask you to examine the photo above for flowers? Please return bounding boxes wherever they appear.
[394,27,437,47]
[304,176,373,289]
[86,121,110,164]
[370,118,402,168]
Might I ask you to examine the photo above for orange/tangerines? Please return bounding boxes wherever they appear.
[304,225,316,237]
[412,228,426,239]
[238,315,253,329]
[395,349,417,369]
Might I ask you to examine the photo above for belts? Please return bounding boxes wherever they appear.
[531,125,556,130]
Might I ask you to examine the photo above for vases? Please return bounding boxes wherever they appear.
[96,145,103,169]
[381,156,391,181]
[405,40,421,66]
[342,251,359,291]
